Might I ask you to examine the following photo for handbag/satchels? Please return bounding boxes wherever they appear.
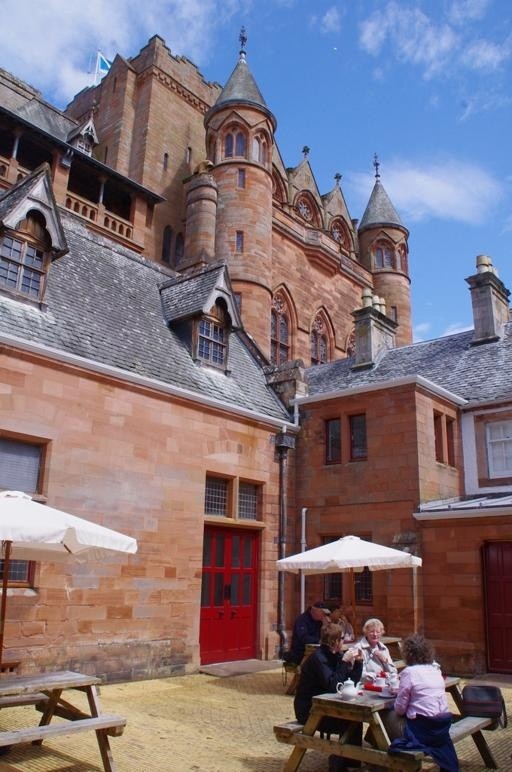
[463,685,502,718]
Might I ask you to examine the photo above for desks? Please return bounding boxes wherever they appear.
[0,671,115,772]
[284,636,406,695]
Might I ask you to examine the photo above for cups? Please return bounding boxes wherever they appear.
[382,688,394,696]
[373,677,385,685]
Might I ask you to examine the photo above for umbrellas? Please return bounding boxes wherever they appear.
[275,535,423,617]
[0,489,139,671]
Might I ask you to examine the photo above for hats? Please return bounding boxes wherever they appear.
[325,598,339,609]
[314,601,331,614]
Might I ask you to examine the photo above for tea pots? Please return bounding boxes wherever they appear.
[335,676,362,700]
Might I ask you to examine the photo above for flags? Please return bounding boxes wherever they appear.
[98,51,111,74]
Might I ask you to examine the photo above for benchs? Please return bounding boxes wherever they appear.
[0,694,127,772]
[274,659,508,772]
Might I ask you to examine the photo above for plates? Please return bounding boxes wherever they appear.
[377,693,396,699]
[373,683,388,688]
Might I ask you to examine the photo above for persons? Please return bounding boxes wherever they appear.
[290,601,330,665]
[363,632,453,751]
[348,618,396,682]
[326,603,355,643]
[293,624,364,770]
